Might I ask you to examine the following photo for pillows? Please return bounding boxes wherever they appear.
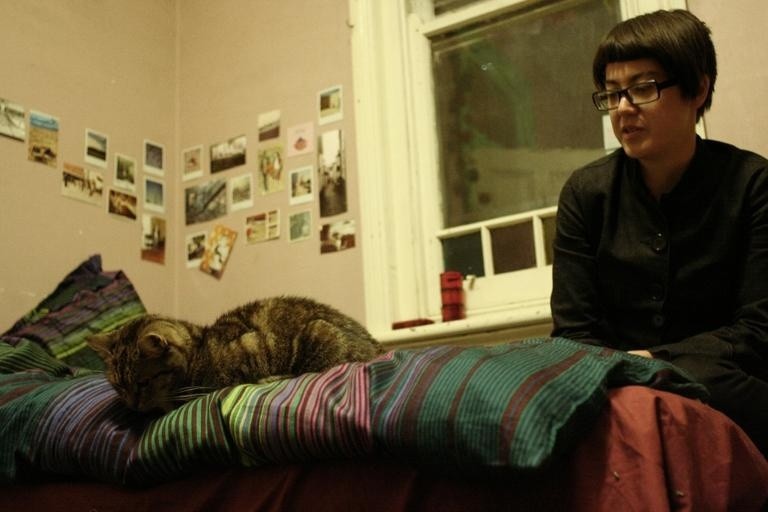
[1,254,150,371]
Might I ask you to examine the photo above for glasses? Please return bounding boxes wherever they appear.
[592,80,677,112]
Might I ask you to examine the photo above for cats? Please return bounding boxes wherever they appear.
[83,296,388,414]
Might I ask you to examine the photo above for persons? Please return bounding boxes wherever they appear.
[331,231,349,251]
[270,152,283,189]
[151,223,162,255]
[258,149,272,192]
[210,235,231,275]
[548,7,768,448]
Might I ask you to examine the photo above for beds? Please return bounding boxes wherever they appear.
[1,332,737,510]
